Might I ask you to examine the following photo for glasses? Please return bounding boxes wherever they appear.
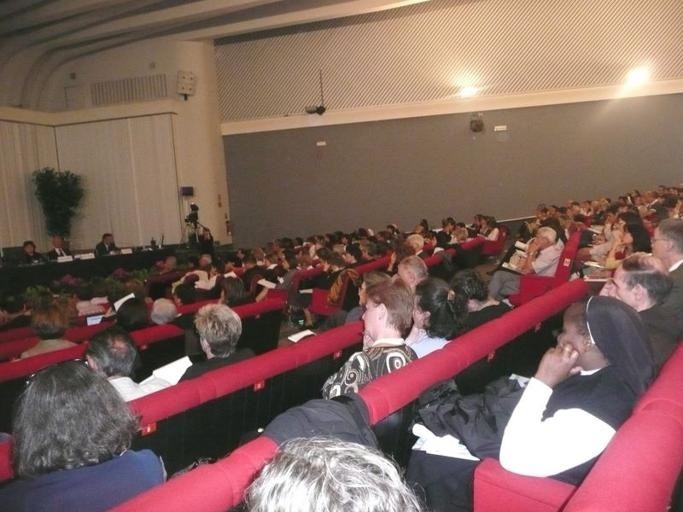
[651,237,668,245]
[608,281,618,289]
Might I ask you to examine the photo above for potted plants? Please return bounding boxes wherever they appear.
[30,167,85,249]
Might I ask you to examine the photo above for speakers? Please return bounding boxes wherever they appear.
[181,187,193,196]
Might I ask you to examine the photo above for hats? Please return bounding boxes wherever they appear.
[536,203,548,212]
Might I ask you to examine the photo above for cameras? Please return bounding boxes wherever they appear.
[184,203,199,222]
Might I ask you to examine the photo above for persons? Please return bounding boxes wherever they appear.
[1,184,682,512]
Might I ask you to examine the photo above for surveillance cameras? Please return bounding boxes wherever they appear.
[305,105,326,114]
